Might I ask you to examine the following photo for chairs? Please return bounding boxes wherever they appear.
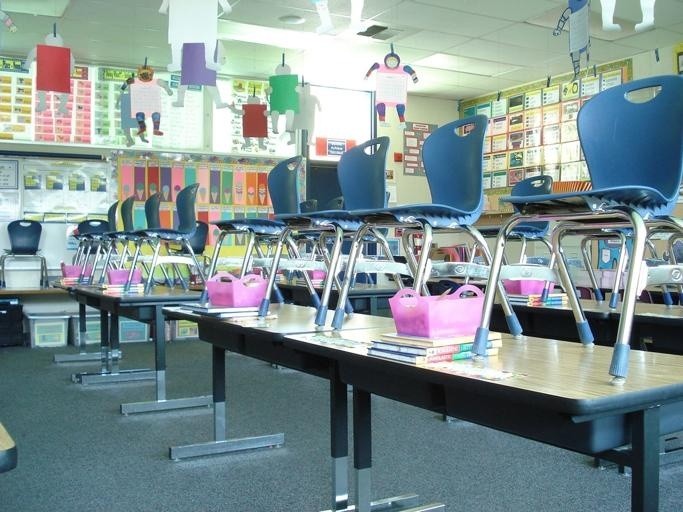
[471,74,683,378]
[1,220,48,291]
[73,183,210,295]
[206,113,524,338]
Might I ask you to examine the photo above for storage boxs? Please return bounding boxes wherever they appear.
[25,309,101,347]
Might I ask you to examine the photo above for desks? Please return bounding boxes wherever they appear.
[50,279,679,512]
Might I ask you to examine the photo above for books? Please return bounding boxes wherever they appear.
[179,300,279,322]
[506,292,569,306]
[54,275,90,286]
[96,283,145,296]
[366,331,502,365]
[274,272,338,289]
